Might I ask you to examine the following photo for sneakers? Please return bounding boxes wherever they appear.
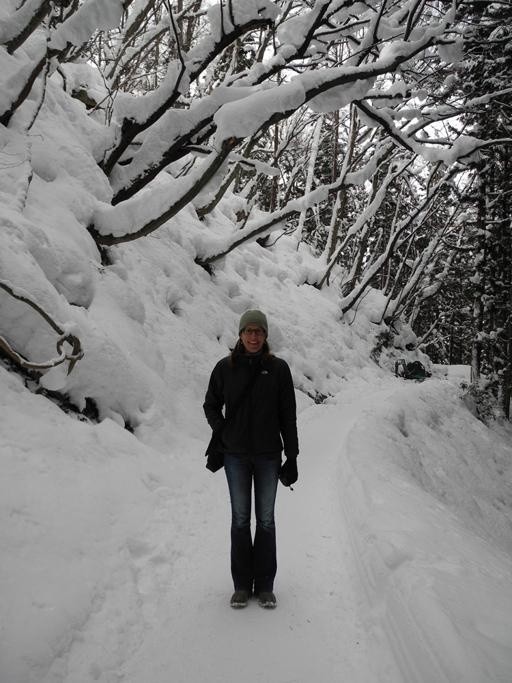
[254,591,276,606]
[231,590,252,608]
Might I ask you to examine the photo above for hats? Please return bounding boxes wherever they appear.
[239,310,268,338]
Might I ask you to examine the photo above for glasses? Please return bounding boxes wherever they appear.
[244,327,263,335]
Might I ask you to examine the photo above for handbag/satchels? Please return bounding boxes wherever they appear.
[205,440,224,472]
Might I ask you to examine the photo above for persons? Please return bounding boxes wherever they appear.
[203,310,299,609]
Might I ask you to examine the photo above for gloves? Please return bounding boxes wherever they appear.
[280,460,298,486]
